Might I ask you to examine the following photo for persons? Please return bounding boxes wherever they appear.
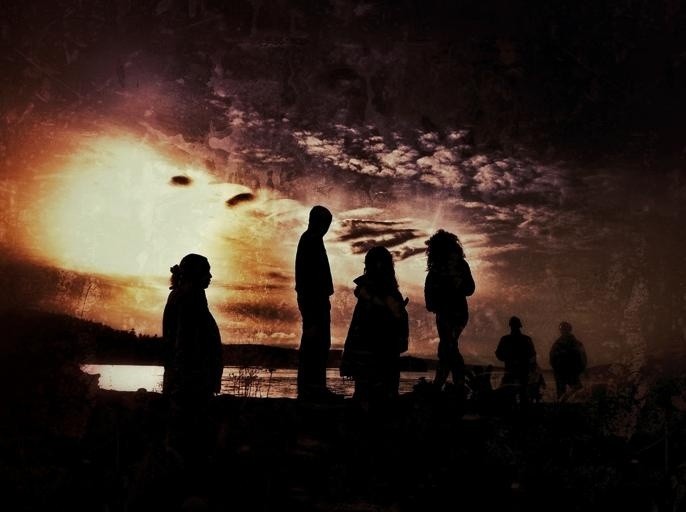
[160,252,226,395]
[531,366,545,404]
[493,316,535,407]
[421,228,472,392]
[549,319,586,396]
[292,201,340,400]
[340,244,409,407]
